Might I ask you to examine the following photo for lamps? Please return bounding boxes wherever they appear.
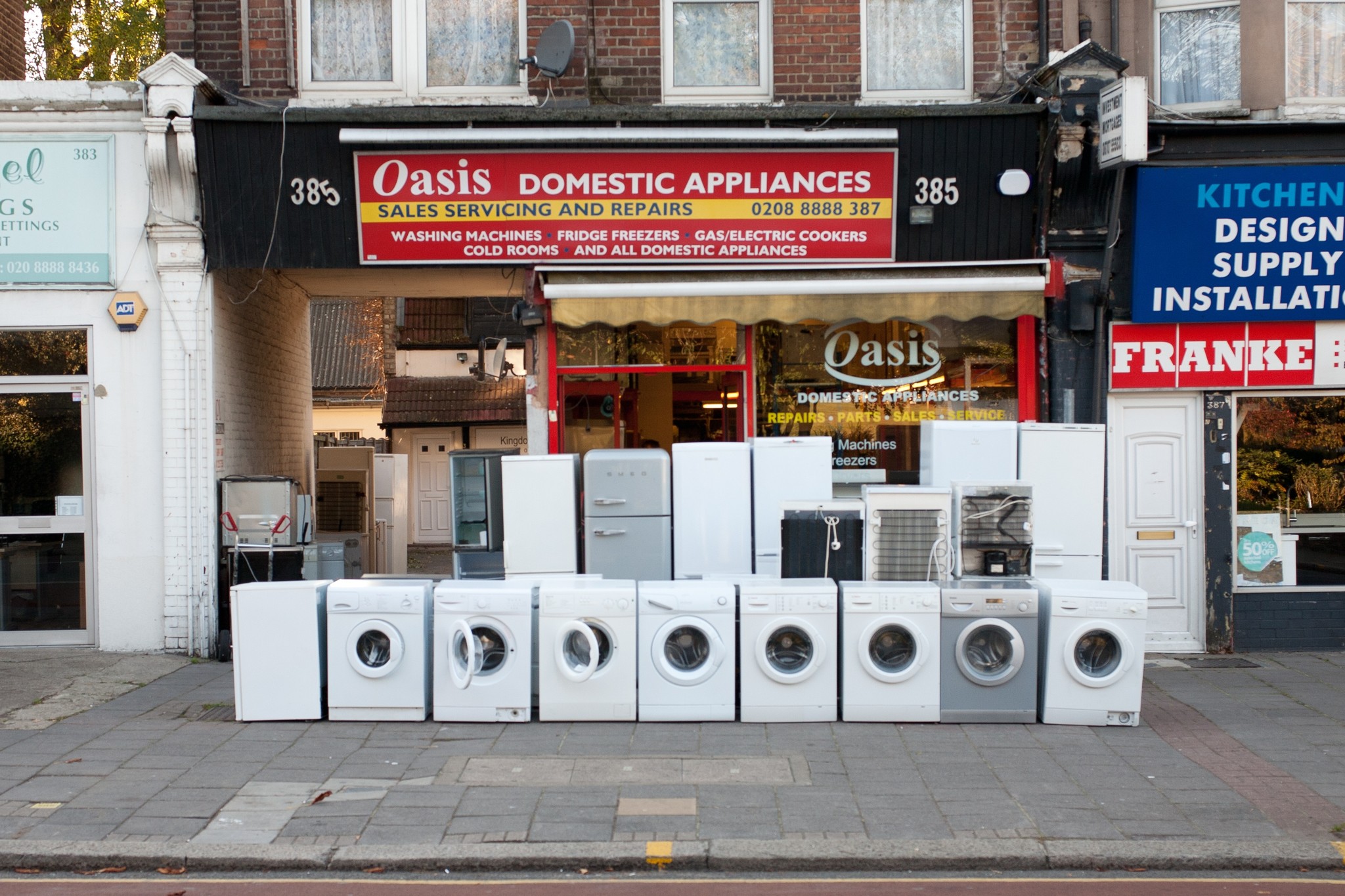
[457,353,468,363]
[519,303,545,327]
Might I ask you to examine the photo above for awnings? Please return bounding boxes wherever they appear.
[532,266,1045,329]
